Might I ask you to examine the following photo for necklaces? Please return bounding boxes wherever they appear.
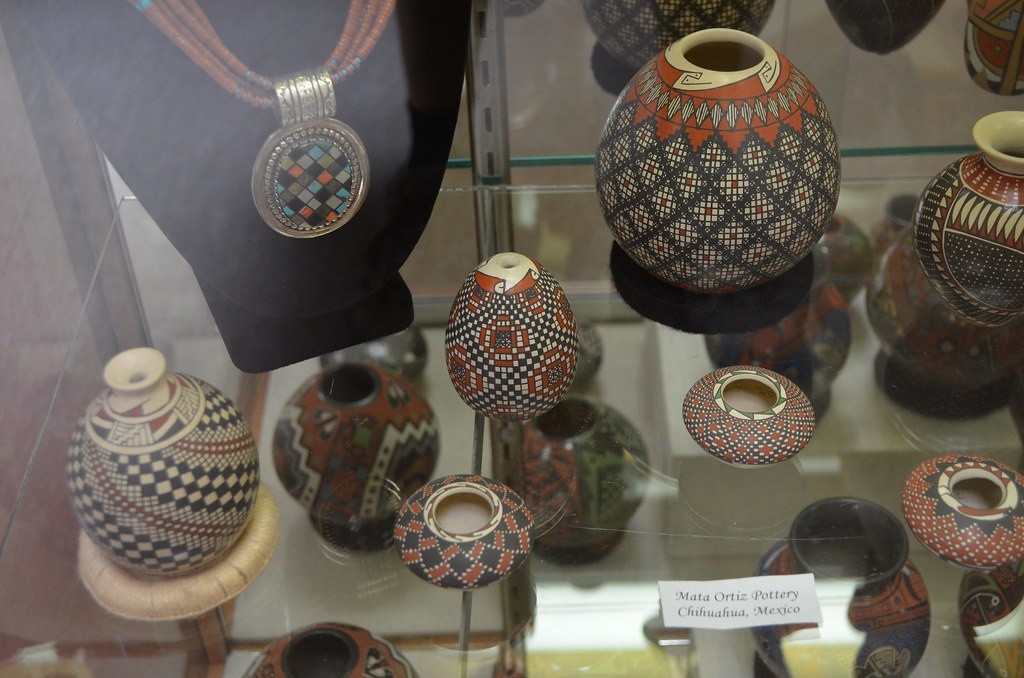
[135,0,393,239]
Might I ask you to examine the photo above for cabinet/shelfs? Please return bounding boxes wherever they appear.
[0,0,1024,678]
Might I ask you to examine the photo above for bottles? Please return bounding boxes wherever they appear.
[864,225,1023,421]
[444,252,577,422]
[752,496,930,678]
[867,193,923,283]
[810,216,873,299]
[237,623,424,678]
[705,279,853,419]
[911,106,1024,327]
[512,395,650,566]
[272,362,438,552]
[596,27,841,294]
[69,347,260,579]
